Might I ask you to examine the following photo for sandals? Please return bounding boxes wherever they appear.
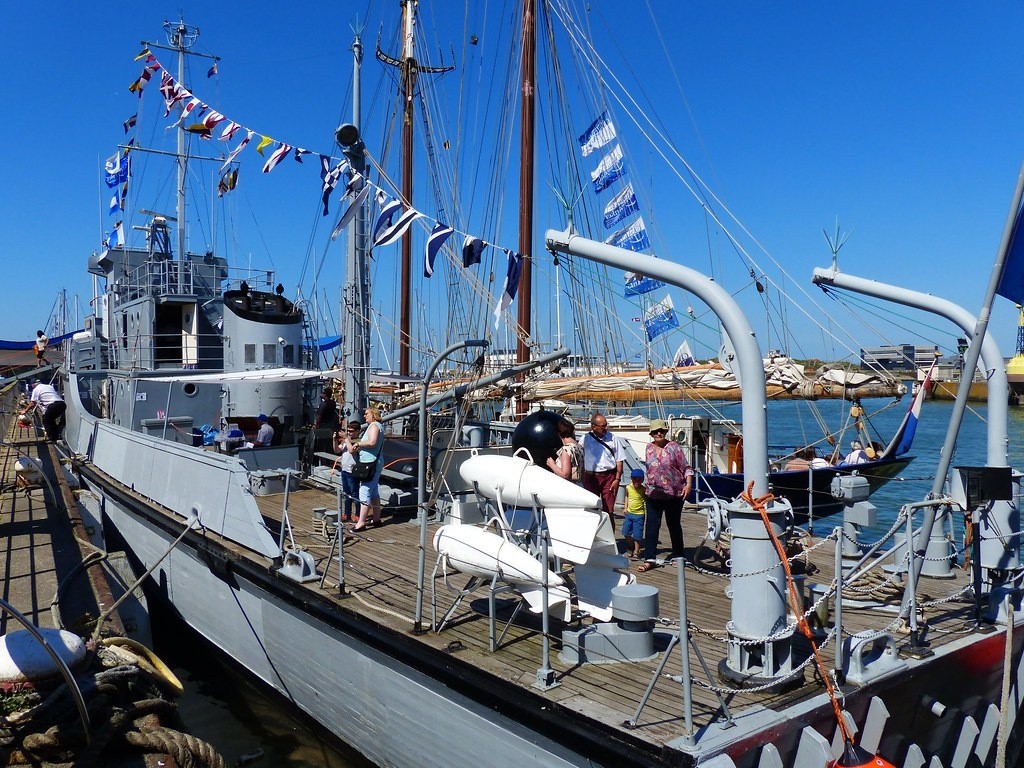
[672,560,678,568]
[638,562,654,572]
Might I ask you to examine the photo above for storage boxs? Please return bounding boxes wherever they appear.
[252,469,302,495]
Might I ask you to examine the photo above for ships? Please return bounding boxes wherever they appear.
[47,0,1024,768]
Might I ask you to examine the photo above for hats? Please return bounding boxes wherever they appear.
[850,441,861,450]
[28,377,40,386]
[649,419,668,434]
[630,469,644,478]
[255,414,268,422]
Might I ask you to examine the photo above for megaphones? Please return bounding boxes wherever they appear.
[278,337,287,347]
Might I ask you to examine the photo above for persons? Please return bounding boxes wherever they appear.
[784,441,884,472]
[251,414,274,447]
[333,420,363,523]
[34,330,51,370]
[311,387,340,467]
[546,419,584,488]
[18,378,67,445]
[350,406,385,533]
[577,414,627,536]
[622,469,647,562]
[637,419,695,572]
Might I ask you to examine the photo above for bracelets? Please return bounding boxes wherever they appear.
[615,478,621,482]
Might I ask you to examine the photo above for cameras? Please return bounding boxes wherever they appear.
[335,432,342,439]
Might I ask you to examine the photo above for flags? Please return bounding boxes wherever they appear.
[99,42,524,333]
[994,202,1024,307]
[577,107,680,342]
[886,363,934,461]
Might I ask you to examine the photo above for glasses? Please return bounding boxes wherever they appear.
[591,422,608,430]
[347,429,356,433]
[651,429,666,434]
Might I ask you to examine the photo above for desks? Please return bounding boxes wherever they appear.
[313,452,414,480]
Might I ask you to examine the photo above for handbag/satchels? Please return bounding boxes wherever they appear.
[33,344,39,355]
[567,447,579,480]
[351,462,376,482]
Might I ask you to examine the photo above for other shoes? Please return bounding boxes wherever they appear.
[46,362,50,366]
[57,434,62,440]
[341,514,351,522]
[350,515,359,525]
[622,549,633,558]
[348,525,367,533]
[45,439,56,443]
[631,553,639,561]
[35,366,41,369]
[365,518,381,526]
